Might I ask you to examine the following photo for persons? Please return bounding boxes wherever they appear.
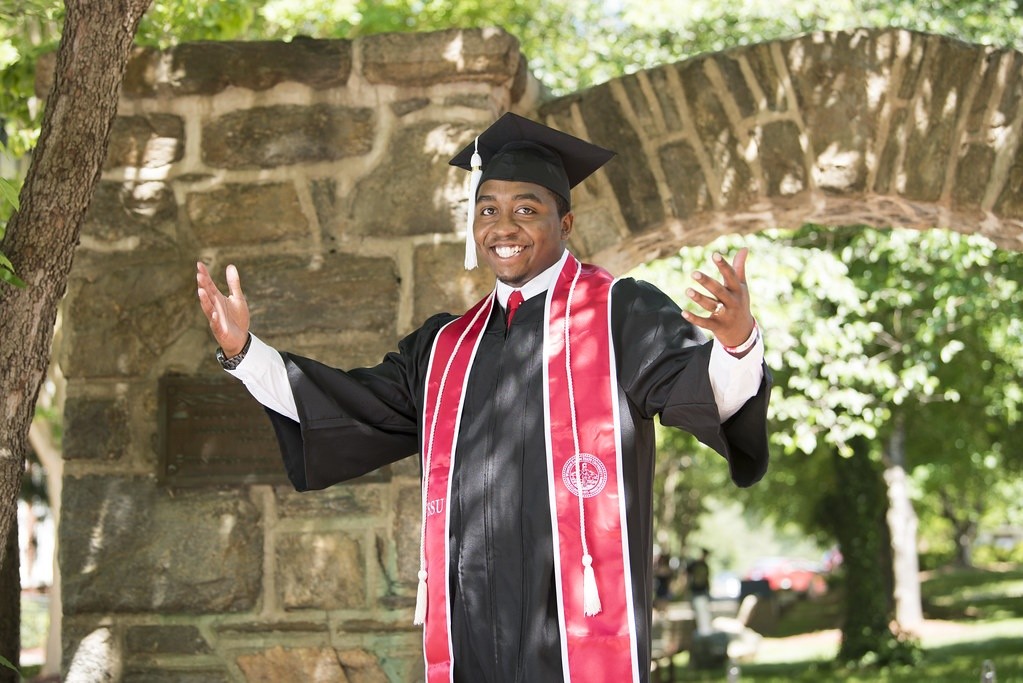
[192,113,772,683]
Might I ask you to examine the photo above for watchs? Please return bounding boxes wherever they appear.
[214,332,252,372]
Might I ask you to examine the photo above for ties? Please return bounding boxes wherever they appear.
[507,290,524,328]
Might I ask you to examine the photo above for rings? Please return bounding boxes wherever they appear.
[714,302,726,316]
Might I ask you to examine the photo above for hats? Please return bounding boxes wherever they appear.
[448,112,617,271]
